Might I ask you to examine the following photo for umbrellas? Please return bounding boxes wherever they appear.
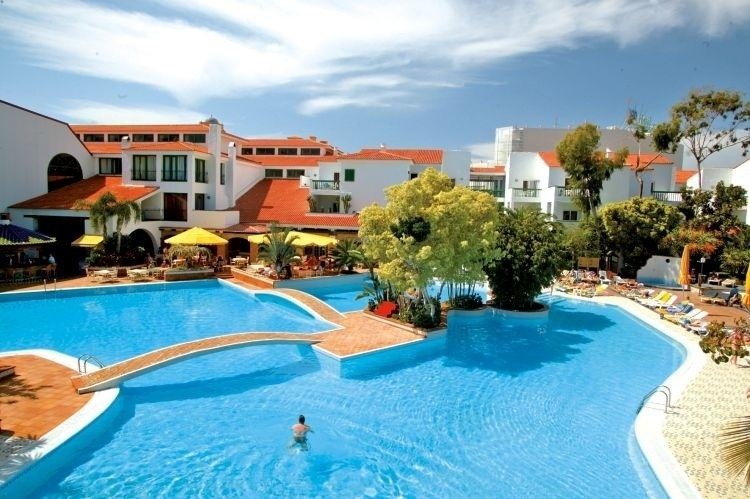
[678,245,690,301]
[247,230,341,248]
[164,226,230,245]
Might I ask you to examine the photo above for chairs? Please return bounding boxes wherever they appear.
[85,256,249,283]
[560,270,719,334]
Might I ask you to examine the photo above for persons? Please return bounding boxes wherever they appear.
[726,291,739,307]
[553,267,648,298]
[289,415,311,452]
[1,246,334,282]
